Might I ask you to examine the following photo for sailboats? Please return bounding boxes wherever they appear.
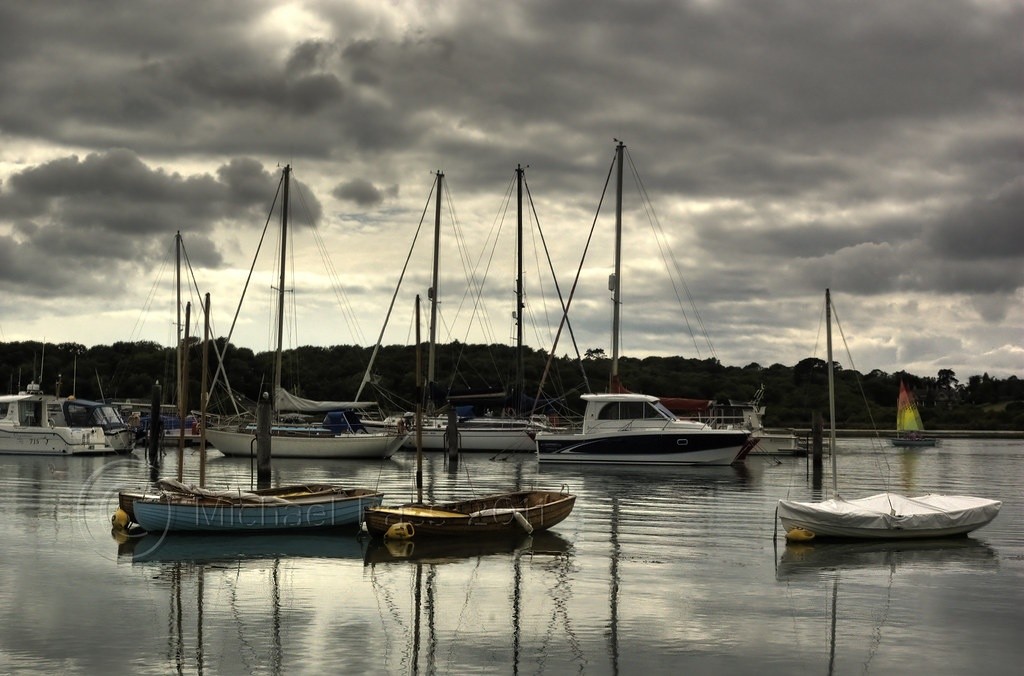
[889,374,938,447]
[118,158,384,539]
[121,142,796,470]
[778,287,1004,541]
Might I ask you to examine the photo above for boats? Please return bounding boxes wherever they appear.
[0,390,135,455]
[364,296,575,541]
